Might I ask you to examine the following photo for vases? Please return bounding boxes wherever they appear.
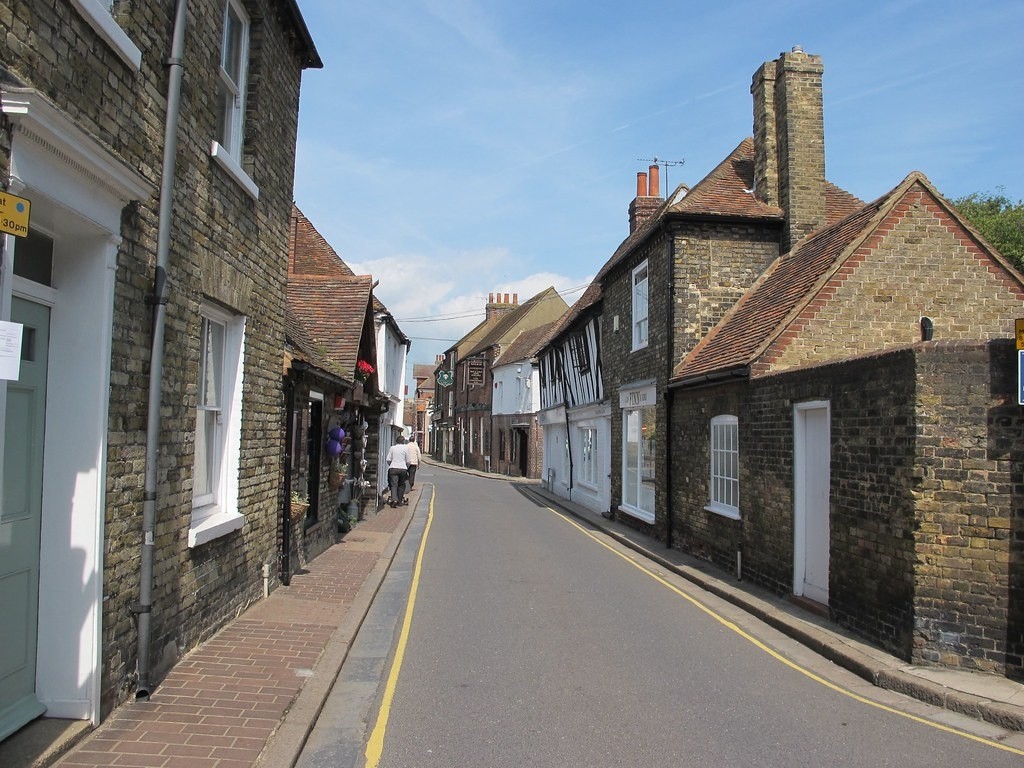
[355,379,364,388]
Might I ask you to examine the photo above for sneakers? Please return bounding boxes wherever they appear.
[391,500,397,508]
[398,503,403,507]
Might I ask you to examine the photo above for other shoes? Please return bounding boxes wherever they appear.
[411,487,413,490]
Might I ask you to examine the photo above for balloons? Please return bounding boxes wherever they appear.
[326,439,341,456]
[329,428,345,441]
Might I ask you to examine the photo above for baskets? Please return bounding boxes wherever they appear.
[329,462,347,489]
[290,492,311,527]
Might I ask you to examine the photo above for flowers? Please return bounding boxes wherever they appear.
[355,360,375,382]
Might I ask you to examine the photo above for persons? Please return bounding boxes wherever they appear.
[386,436,411,508]
[406,436,421,490]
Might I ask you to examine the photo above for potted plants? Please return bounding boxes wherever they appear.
[330,459,349,487]
[348,499,360,519]
[290,491,311,528]
